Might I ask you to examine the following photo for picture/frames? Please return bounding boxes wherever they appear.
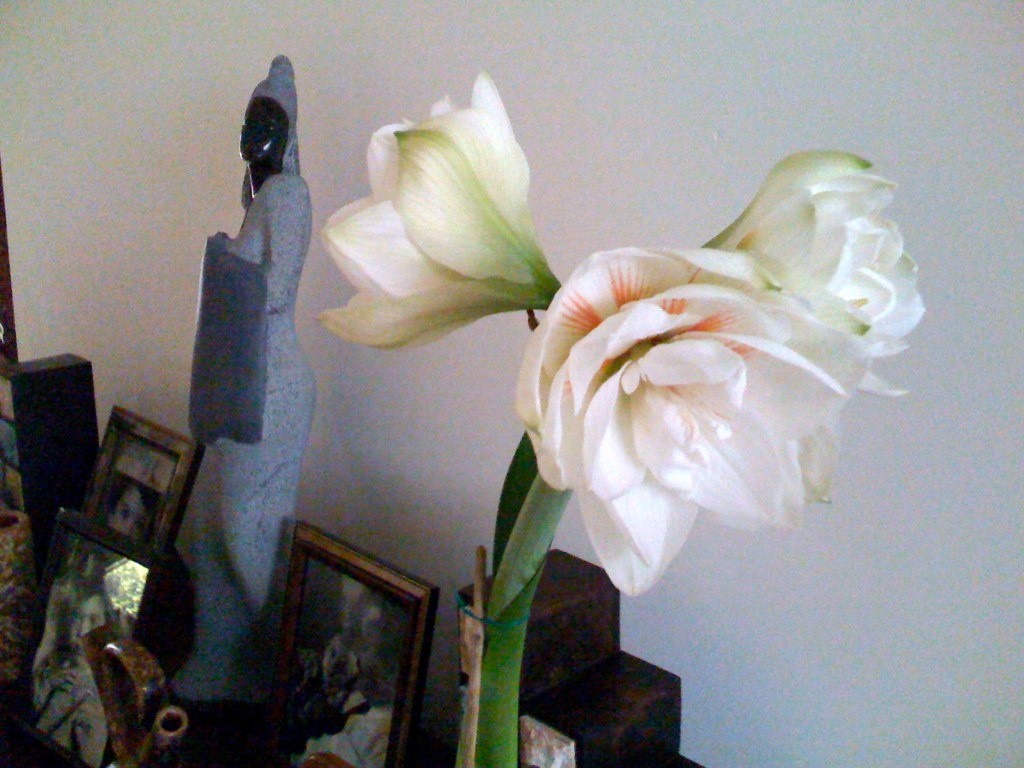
[73,404,204,557]
[13,505,177,768]
[256,523,441,767]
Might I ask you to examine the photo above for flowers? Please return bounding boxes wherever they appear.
[315,71,926,768]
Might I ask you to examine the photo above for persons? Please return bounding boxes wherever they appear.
[31,583,116,768]
[294,627,375,743]
[100,475,161,541]
[169,53,314,697]
